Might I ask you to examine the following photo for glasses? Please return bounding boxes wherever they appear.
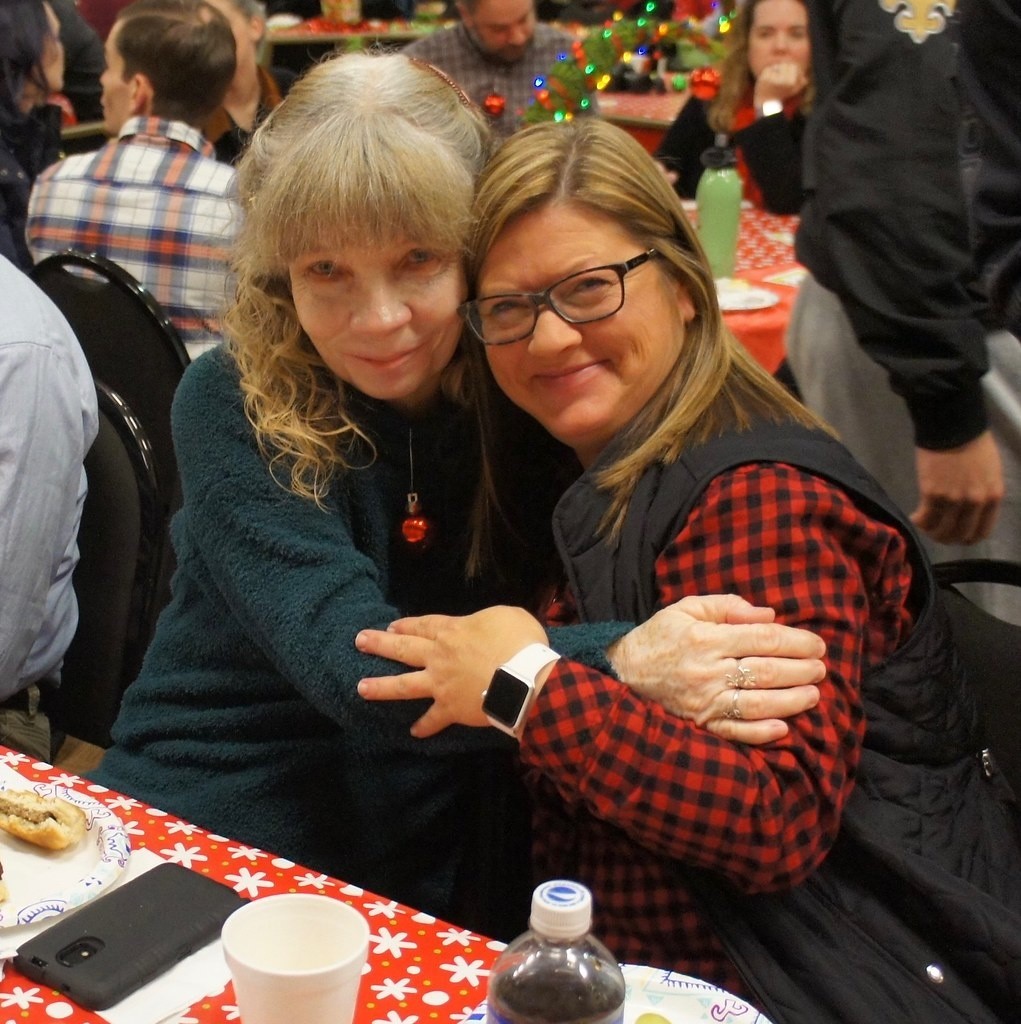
[457,234,681,347]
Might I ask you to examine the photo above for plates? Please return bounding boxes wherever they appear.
[1,780,130,930]
[462,961,774,1024]
[713,279,779,312]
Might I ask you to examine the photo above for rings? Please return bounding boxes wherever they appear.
[727,657,756,687]
[723,689,744,721]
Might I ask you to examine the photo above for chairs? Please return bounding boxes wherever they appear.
[26,244,188,781]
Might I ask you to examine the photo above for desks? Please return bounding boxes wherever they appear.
[0,747,528,1024]
[269,34,419,75]
[599,93,806,374]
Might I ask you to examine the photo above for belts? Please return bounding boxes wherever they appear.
[0,678,53,712]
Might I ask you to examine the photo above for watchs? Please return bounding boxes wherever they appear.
[479,643,562,739]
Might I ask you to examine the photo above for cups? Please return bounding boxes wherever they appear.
[220,892,371,1024]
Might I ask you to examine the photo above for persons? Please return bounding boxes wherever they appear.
[777,0,1021,641]
[404,0,595,145]
[25,0,249,367]
[80,49,825,948]
[0,250,97,715]
[643,0,836,407]
[356,115,1021,1024]
[0,0,307,222]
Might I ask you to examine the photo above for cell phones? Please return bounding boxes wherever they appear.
[13,861,243,1011]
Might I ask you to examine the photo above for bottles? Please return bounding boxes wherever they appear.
[695,132,743,279]
[483,879,626,1024]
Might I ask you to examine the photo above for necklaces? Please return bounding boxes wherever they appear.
[397,418,430,545]
